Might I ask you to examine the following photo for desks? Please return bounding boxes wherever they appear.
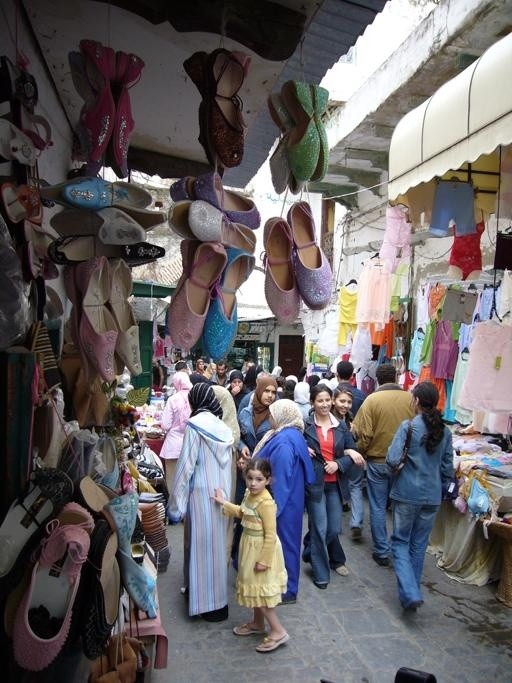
[143,438,164,453]
[426,453,512,606]
[125,553,167,683]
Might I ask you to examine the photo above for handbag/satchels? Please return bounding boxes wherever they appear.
[393,446,408,475]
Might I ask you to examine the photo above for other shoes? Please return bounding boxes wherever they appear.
[2,177,165,425]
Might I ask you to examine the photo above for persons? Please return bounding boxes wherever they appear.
[159,358,367,622]
[350,364,454,609]
[209,461,290,652]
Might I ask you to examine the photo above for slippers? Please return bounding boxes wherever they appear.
[233,624,264,635]
[256,634,290,652]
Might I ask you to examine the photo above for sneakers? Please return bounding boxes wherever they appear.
[315,565,348,589]
[167,172,333,359]
[78,44,145,178]
[184,48,251,169]
[0,438,156,673]
[268,80,329,193]
[351,527,362,540]
[373,552,390,568]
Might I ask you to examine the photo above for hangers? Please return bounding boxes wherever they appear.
[500,227,511,235]
[363,373,373,381]
[439,174,472,183]
[361,253,380,263]
[416,272,512,362]
[334,279,356,291]
